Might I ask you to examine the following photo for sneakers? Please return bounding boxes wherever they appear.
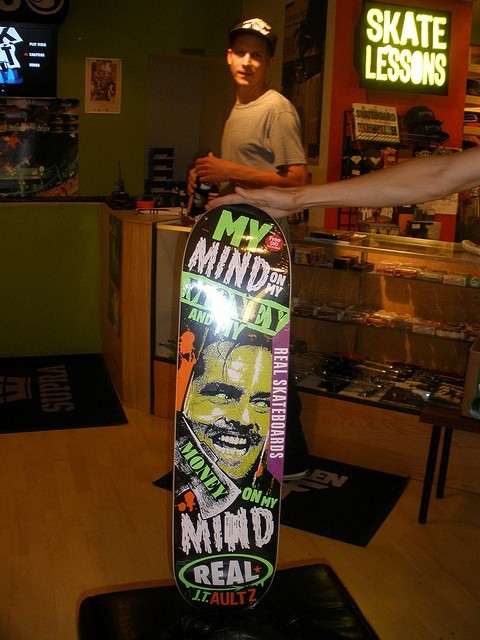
[283,457,309,482]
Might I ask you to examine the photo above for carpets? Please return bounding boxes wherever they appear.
[154,451,411,547]
[75,558,379,638]
[0,352,129,434]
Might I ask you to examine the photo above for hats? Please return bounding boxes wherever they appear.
[404,105,444,126]
[409,123,449,141]
[228,17,276,56]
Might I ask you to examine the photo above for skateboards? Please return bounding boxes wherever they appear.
[171,202,293,618]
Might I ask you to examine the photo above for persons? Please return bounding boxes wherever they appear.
[182,327,274,485]
[92,60,113,94]
[205,140,480,222]
[186,15,310,482]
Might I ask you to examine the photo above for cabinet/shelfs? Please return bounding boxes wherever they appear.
[99,198,479,495]
[336,104,449,235]
